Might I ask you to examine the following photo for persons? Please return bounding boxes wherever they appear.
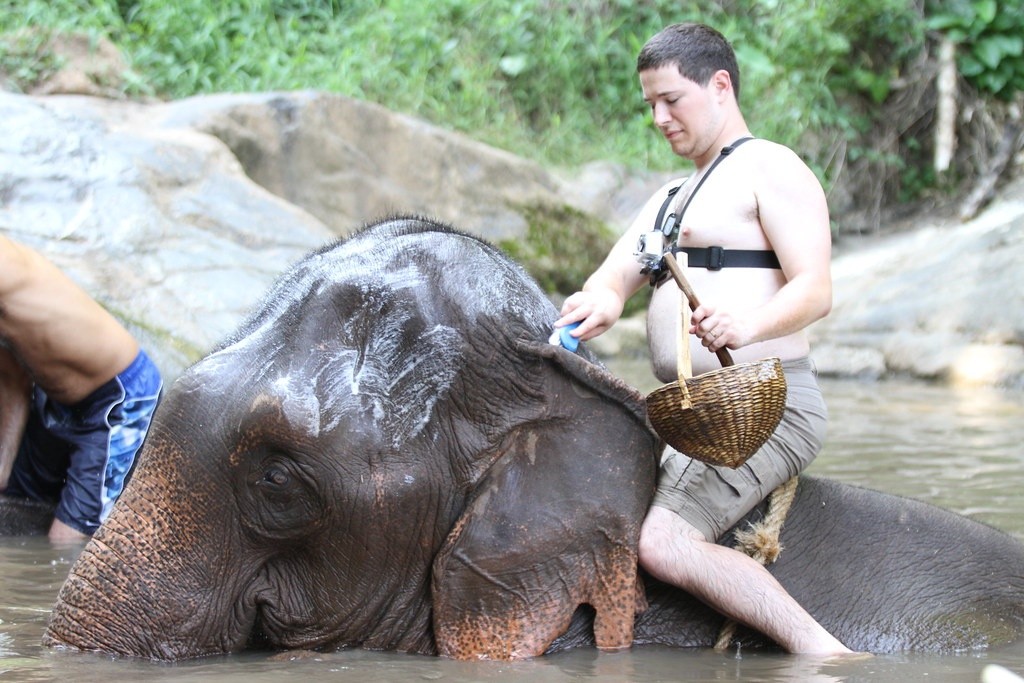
[554,23,855,654]
[0,235,161,543]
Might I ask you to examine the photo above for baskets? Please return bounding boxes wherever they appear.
[645,251,787,470]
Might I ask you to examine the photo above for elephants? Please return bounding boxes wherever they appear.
[39,215,1023,663]
